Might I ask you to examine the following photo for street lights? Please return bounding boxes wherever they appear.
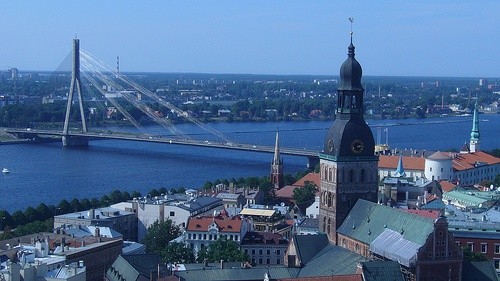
[158,262,166,279]
[55,208,60,216]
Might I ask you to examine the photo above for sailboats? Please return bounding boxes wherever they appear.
[374,127,391,156]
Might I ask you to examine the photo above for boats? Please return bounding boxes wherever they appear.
[2,168,10,174]
